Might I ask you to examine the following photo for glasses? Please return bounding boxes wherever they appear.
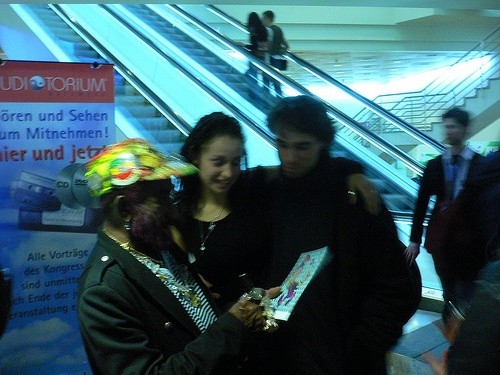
[136,190,183,207]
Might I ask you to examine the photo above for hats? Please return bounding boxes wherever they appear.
[84,139,202,196]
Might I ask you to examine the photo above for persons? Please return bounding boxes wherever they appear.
[262,11,287,98]
[236,12,269,98]
[247,96,403,375]
[171,111,382,375]
[423,172,500,375]
[77,138,282,375]
[404,109,490,315]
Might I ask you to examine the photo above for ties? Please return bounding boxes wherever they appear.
[450,154,460,196]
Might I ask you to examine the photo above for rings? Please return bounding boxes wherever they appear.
[260,300,278,333]
[348,191,355,195]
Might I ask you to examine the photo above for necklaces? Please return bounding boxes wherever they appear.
[198,205,224,251]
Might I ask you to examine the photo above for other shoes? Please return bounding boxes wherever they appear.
[425,209,462,254]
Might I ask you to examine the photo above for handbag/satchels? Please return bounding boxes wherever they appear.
[270,54,287,69]
